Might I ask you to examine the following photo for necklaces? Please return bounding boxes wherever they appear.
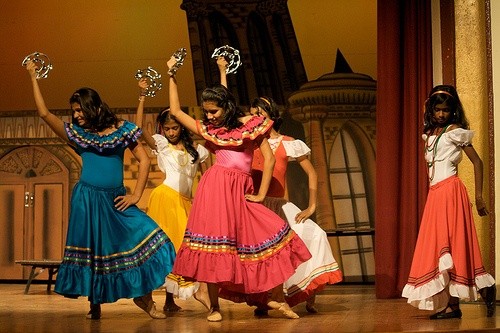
[168,142,189,168]
[423,122,450,181]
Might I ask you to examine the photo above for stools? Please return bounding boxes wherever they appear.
[14,260,62,296]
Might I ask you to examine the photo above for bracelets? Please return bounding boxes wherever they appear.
[138,93,146,101]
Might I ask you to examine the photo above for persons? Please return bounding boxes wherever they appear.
[217,56,343,317]
[167,56,300,321]
[24,61,176,319]
[134,78,209,315]
[402,84,497,320]
